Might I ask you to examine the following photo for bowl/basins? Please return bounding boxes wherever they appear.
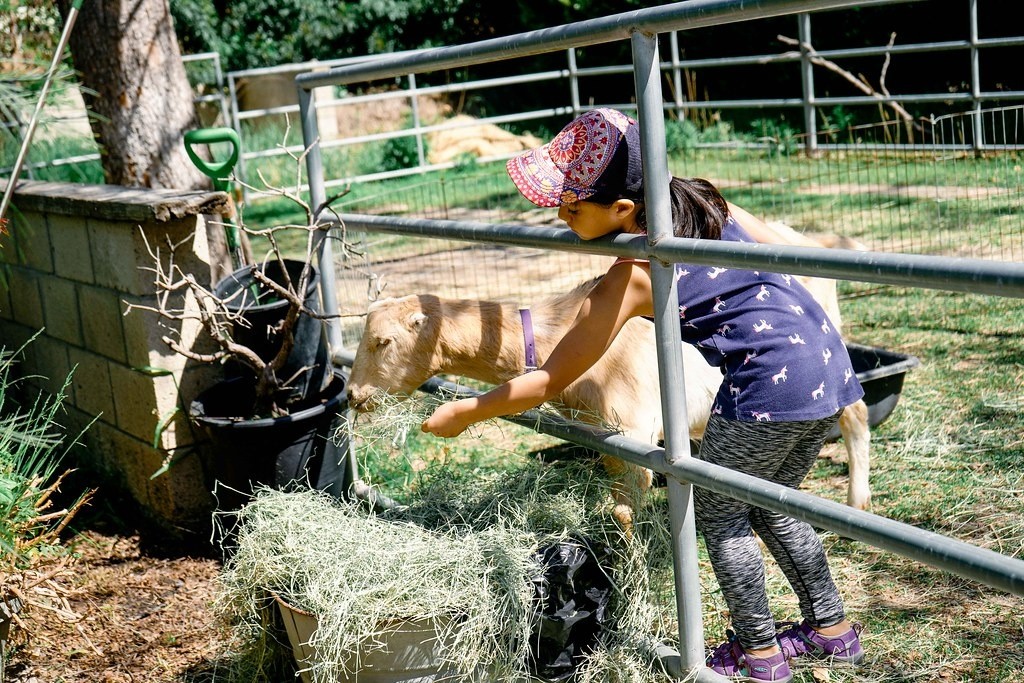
[822,340,915,442]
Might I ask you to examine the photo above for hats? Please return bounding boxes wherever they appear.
[506,108,672,208]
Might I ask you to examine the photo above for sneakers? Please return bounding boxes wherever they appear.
[705,629,794,683]
[775,618,864,665]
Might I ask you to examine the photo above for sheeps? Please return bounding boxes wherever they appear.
[346,224,874,540]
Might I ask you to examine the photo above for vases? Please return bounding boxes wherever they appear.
[214,259,334,401]
[188,370,353,555]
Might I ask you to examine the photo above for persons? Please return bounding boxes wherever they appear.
[419,107,869,682]
